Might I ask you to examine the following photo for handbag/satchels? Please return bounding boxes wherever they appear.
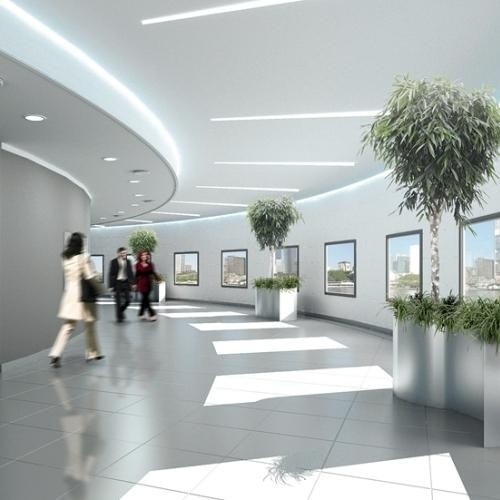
[81,279,96,302]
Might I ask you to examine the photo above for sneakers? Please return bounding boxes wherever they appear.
[114,311,130,323]
[138,315,158,322]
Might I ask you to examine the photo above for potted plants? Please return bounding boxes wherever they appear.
[244,194,304,322]
[121,227,167,304]
[353,72,499,450]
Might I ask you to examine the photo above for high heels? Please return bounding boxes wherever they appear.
[50,356,62,368]
[84,354,106,365]
